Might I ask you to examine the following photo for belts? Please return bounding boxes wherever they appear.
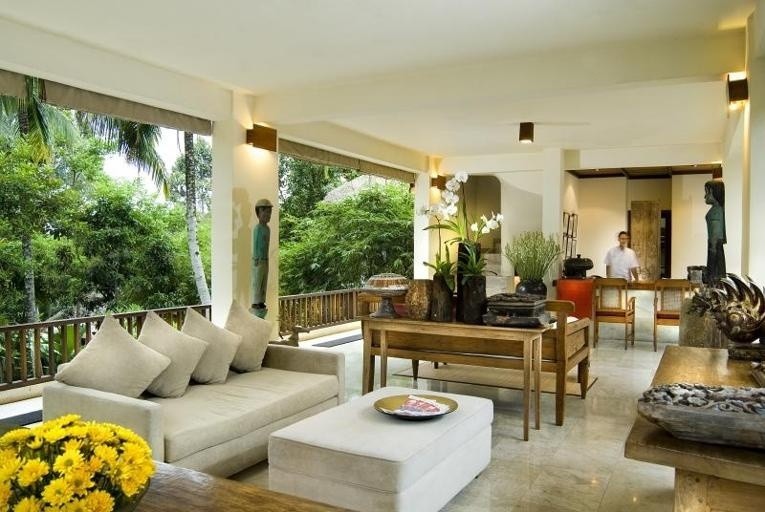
[260,257,267,260]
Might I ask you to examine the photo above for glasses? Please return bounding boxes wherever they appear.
[620,237,631,240]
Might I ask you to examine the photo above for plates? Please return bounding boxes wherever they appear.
[374,394,458,421]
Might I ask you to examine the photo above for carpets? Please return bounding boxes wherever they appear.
[391,361,598,398]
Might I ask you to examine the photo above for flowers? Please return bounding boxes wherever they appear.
[423,180,504,247]
[416,170,469,292]
[2,412,157,511]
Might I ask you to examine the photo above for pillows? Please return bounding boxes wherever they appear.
[52,314,171,397]
[180,304,243,387]
[138,310,211,399]
[226,299,274,375]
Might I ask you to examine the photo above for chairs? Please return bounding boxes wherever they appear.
[652,277,694,353]
[590,276,637,351]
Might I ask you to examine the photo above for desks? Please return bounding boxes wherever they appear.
[126,460,358,512]
[359,313,554,442]
[623,345,765,511]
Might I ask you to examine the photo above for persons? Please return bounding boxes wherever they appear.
[251,199,274,309]
[603,231,641,282]
[703,178,728,277]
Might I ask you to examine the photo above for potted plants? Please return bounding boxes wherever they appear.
[502,230,565,301]
[458,239,498,324]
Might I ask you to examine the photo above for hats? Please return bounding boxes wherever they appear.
[254,198,273,207]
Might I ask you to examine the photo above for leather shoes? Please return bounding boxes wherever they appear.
[251,304,260,309]
[258,303,265,308]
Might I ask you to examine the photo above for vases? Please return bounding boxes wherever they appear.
[454,240,482,323]
[429,274,456,324]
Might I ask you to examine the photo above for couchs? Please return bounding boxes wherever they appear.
[42,342,347,481]
[357,291,593,427]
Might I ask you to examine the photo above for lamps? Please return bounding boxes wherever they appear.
[246,123,277,154]
[518,122,535,145]
[726,75,749,115]
